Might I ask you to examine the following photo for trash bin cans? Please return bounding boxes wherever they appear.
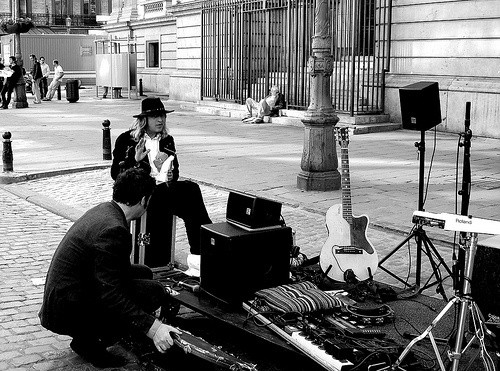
[65,79,79,103]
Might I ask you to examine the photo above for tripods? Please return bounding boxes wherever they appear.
[393,210,500,371]
[378,129,453,303]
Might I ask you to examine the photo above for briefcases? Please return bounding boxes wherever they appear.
[130,210,176,272]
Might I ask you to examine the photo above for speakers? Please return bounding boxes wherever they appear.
[199,190,294,312]
[400,81,442,132]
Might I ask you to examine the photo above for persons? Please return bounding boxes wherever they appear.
[102,87,109,98]
[110,96,215,255]
[29,54,44,104]
[0,56,20,109]
[42,60,64,101]
[38,56,51,99]
[38,167,183,369]
[240,85,286,124]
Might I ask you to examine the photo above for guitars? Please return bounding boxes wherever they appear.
[319,125,378,282]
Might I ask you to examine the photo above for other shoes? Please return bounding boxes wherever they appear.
[34,101,41,104]
[0,104,5,108]
[43,97,51,101]
[70,340,128,368]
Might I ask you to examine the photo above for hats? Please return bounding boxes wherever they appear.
[132,95,175,116]
